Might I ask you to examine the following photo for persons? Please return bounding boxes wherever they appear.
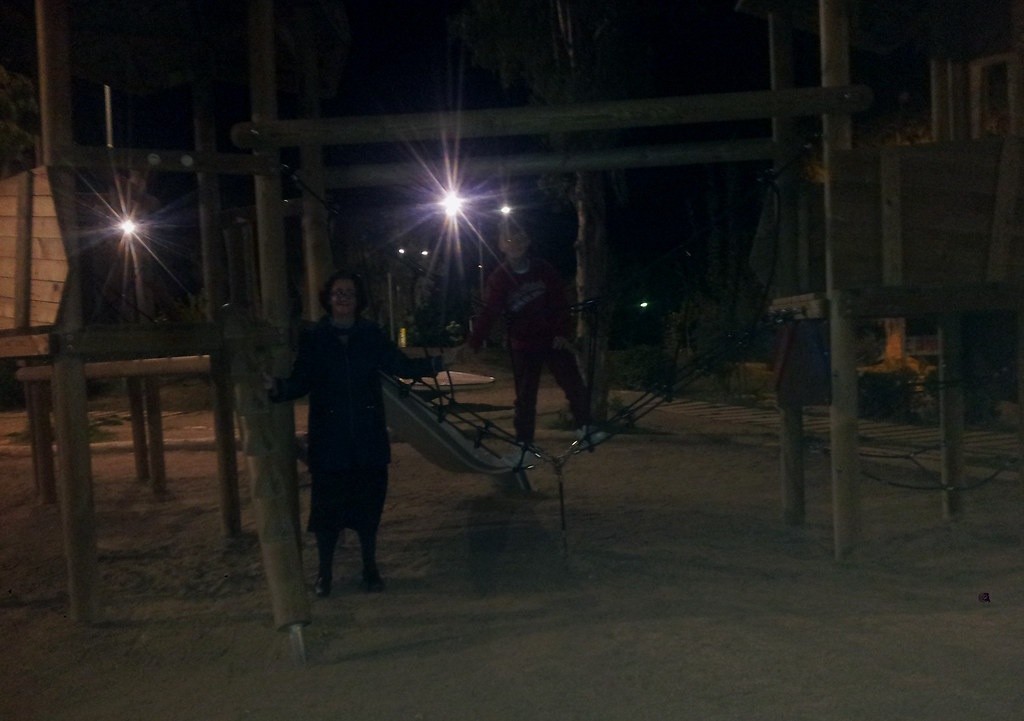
[258,271,467,598]
[457,217,596,445]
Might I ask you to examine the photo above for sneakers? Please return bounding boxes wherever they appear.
[513,443,546,468]
[363,570,384,592]
[314,570,333,596]
[576,424,607,450]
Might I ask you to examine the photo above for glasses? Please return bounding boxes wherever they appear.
[331,289,359,299]
[502,237,527,246]
